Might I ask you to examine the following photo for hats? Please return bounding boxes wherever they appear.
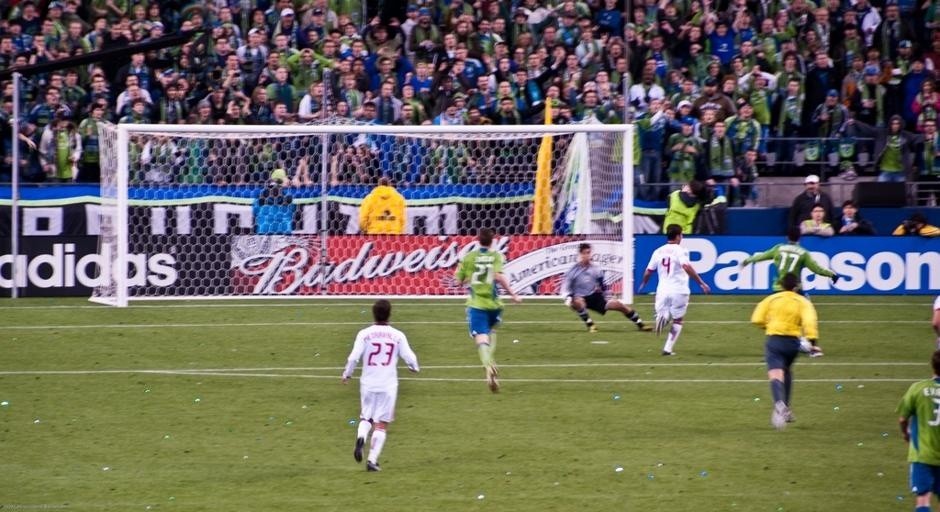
[280,8,294,16]
[804,175,820,184]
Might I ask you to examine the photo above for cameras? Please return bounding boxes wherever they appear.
[904,221,916,230]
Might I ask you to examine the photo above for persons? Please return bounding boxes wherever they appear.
[253,169,297,234]
[891,211,940,238]
[562,243,653,333]
[340,298,421,473]
[0,0,940,207]
[357,178,409,234]
[453,229,520,391]
[740,227,840,357]
[898,349,939,512]
[799,203,835,237]
[786,175,838,225]
[929,288,940,344]
[751,272,818,424]
[663,182,702,234]
[832,200,868,235]
[636,222,709,354]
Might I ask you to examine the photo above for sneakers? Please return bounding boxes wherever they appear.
[810,349,823,357]
[489,364,499,393]
[641,325,653,331]
[354,437,381,470]
[588,324,598,332]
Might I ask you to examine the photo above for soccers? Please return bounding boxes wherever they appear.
[799,336,811,352]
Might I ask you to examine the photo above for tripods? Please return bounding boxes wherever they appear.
[694,207,714,235]
